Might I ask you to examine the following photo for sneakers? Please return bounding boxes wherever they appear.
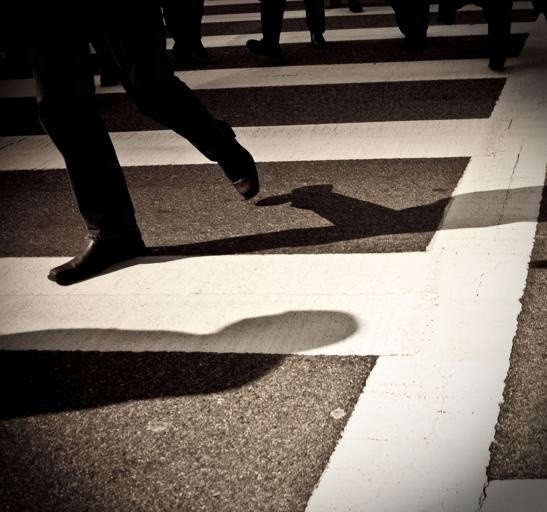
[246,39,283,56]
[310,31,330,49]
[213,119,258,199]
[48,225,147,285]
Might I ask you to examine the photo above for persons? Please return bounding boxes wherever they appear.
[329,1,363,16]
[243,1,327,62]
[160,0,209,63]
[387,1,468,50]
[473,1,547,73]
[20,1,261,285]
